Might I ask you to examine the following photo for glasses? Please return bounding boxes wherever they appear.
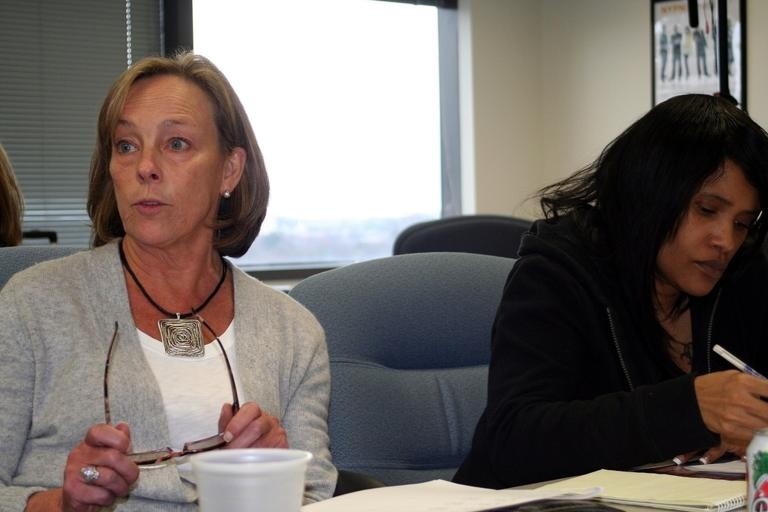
[103,306,240,466]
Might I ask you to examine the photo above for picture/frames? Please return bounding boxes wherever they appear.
[649,0,747,110]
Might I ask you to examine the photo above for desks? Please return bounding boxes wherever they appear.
[306,454,748,512]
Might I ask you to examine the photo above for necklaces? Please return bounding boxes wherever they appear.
[118,234,228,358]
[661,324,692,366]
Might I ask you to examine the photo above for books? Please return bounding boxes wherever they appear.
[302,456,749,512]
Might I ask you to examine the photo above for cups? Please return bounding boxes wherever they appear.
[189,448,313,512]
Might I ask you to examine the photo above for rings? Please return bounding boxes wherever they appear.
[79,464,100,481]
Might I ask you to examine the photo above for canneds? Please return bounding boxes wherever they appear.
[747,429,768,512]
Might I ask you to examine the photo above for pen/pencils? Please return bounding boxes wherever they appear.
[712,343,767,379]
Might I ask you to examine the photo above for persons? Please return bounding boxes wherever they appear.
[450,92,767,489]
[0,145,27,247]
[0,44,340,512]
[658,16,735,82]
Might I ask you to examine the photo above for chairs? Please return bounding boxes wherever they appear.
[289,253,516,486]
[392,213,531,260]
[1,246,90,290]
